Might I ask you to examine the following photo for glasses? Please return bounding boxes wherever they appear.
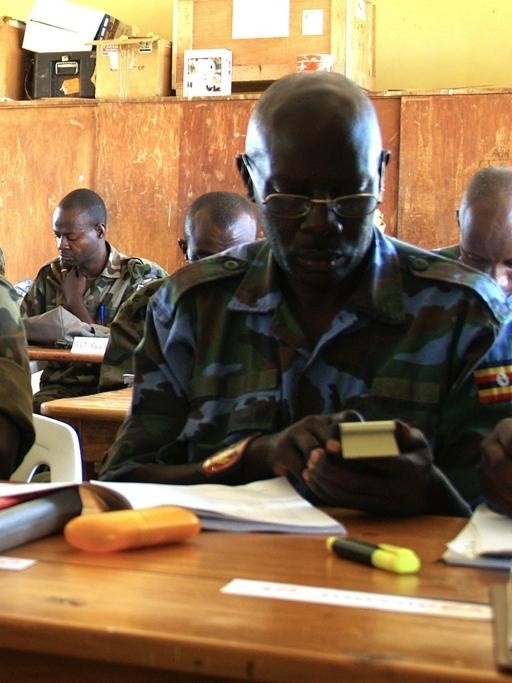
[241,150,385,219]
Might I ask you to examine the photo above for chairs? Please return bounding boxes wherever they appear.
[9,412,83,484]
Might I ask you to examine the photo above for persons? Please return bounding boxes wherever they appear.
[19,184,168,483]
[425,163,512,307]
[467,417,512,525]
[93,69,511,520]
[1,274,37,482]
[179,189,259,266]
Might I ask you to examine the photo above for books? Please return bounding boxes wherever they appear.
[0,473,348,536]
[442,501,511,570]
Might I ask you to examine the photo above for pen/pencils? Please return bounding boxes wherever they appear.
[326,535,420,573]
[100,304,106,325]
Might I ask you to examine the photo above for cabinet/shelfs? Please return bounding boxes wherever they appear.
[0,86,511,283]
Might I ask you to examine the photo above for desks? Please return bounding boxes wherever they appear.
[27,336,110,362]
[1,509,511,682]
[40,385,134,463]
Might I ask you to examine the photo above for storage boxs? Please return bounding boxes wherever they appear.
[21,0,142,52]
[172,0,376,95]
[1,14,31,104]
[86,37,173,99]
